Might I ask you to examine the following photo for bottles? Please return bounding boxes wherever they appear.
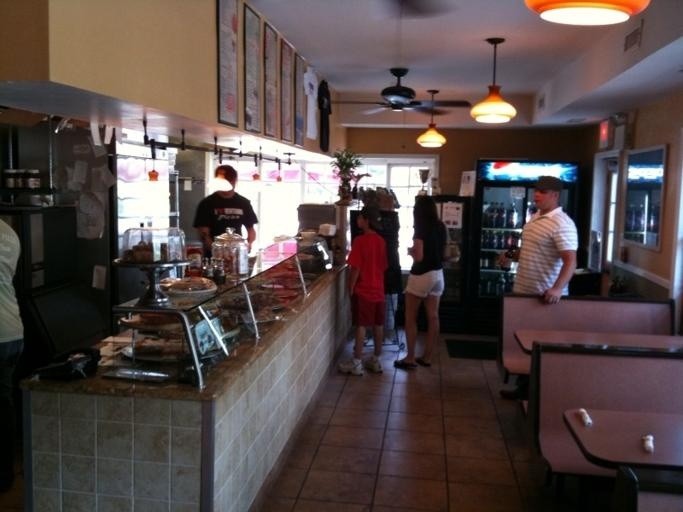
[479,200,533,297]
[5,168,41,193]
[626,203,658,233]
[184,228,248,285]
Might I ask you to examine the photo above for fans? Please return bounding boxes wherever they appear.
[328,68,471,118]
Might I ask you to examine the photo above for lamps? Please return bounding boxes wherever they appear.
[524,0,650,25]
[470,41,517,124]
[416,95,447,147]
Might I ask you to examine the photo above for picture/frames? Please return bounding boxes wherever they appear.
[215,1,304,147]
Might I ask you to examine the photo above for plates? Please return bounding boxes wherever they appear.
[120,271,317,361]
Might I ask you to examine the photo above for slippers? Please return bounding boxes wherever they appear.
[394,359,418,367]
[415,358,432,367]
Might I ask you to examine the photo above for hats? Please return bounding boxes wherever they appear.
[362,204,384,232]
[531,177,563,189]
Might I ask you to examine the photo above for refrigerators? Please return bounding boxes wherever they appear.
[468,159,584,336]
[624,163,664,248]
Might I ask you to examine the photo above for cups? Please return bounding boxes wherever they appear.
[301,231,316,242]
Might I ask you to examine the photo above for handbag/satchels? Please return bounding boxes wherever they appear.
[384,264,403,295]
[443,241,461,263]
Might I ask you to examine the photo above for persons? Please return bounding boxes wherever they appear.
[192,165,257,251]
[394,195,447,369]
[499,175,580,403]
[338,203,390,376]
[0,217,26,493]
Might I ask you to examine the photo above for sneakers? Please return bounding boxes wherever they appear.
[365,355,384,373]
[338,358,364,375]
[500,387,529,400]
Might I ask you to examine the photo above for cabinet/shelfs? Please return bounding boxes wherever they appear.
[1,109,58,213]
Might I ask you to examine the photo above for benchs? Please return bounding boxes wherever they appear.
[500,291,683,416]
[606,471,683,512]
[529,340,683,508]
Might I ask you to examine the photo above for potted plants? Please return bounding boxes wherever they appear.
[328,143,364,192]
